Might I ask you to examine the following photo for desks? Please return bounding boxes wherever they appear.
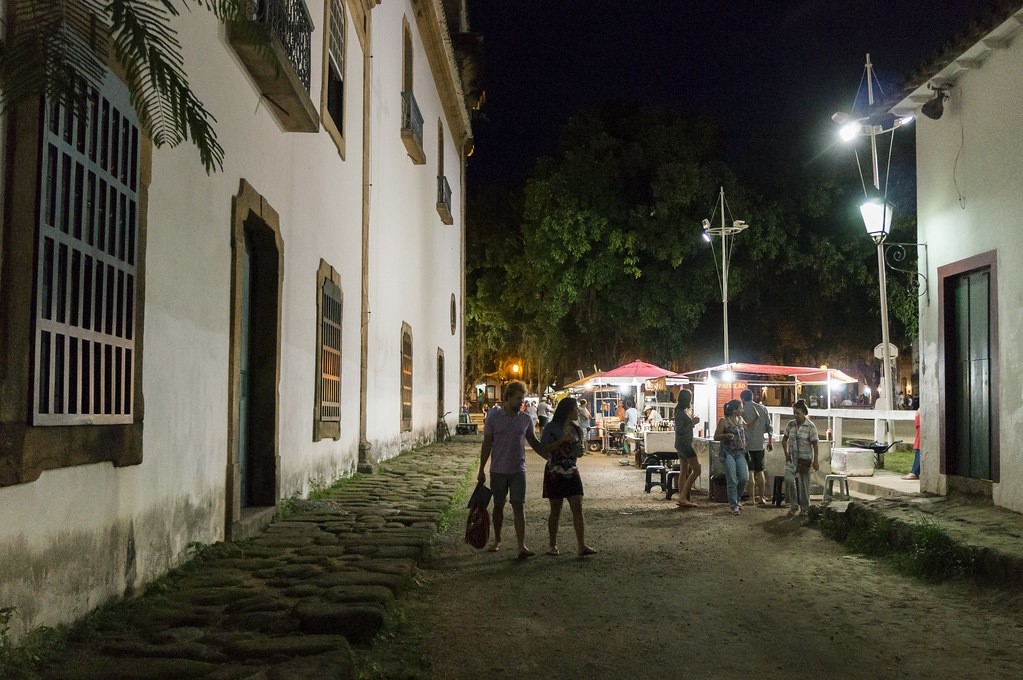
[633,437,644,468]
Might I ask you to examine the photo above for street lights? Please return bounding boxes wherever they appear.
[838,54,914,450]
[513,365,519,380]
[545,369,549,393]
[702,186,750,366]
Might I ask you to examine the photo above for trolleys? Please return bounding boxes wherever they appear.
[846,439,903,469]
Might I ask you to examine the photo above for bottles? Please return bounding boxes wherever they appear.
[649,421,672,431]
[826,428,832,441]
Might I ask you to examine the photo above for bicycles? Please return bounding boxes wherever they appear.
[437,412,452,443]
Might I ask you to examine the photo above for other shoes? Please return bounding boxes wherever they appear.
[732,501,743,515]
[901,473,919,480]
[745,498,765,506]
[787,507,809,516]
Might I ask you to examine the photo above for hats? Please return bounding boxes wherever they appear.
[541,397,547,400]
[643,405,651,411]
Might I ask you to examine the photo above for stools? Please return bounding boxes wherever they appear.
[822,474,850,503]
[666,470,690,500]
[645,465,667,493]
[771,475,801,507]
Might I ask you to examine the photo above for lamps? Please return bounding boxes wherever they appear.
[921,84,947,119]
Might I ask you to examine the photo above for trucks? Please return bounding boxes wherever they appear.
[471,383,487,403]
[525,397,548,410]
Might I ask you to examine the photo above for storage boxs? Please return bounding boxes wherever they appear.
[644,430,677,453]
[604,416,621,428]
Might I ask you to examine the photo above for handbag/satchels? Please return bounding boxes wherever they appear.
[465,483,490,549]
[745,451,751,463]
[795,458,812,474]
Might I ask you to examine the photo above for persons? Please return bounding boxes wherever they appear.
[832,390,870,407]
[623,400,637,454]
[740,389,773,506]
[901,409,920,480]
[578,399,594,456]
[463,400,473,423]
[643,405,663,423]
[672,389,703,507]
[478,379,549,558]
[713,399,760,515]
[781,398,820,519]
[540,397,598,556]
[615,401,625,420]
[897,390,919,410]
[483,394,557,433]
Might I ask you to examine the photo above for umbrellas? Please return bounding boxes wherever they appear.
[563,371,607,425]
[591,358,689,426]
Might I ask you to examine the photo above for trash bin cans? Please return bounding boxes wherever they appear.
[711,474,728,503]
[673,464,692,488]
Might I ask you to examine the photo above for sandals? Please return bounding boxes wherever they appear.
[578,545,597,556]
[547,546,560,555]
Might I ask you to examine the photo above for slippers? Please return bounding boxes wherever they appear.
[489,542,502,552]
[517,545,536,558]
[675,502,698,508]
